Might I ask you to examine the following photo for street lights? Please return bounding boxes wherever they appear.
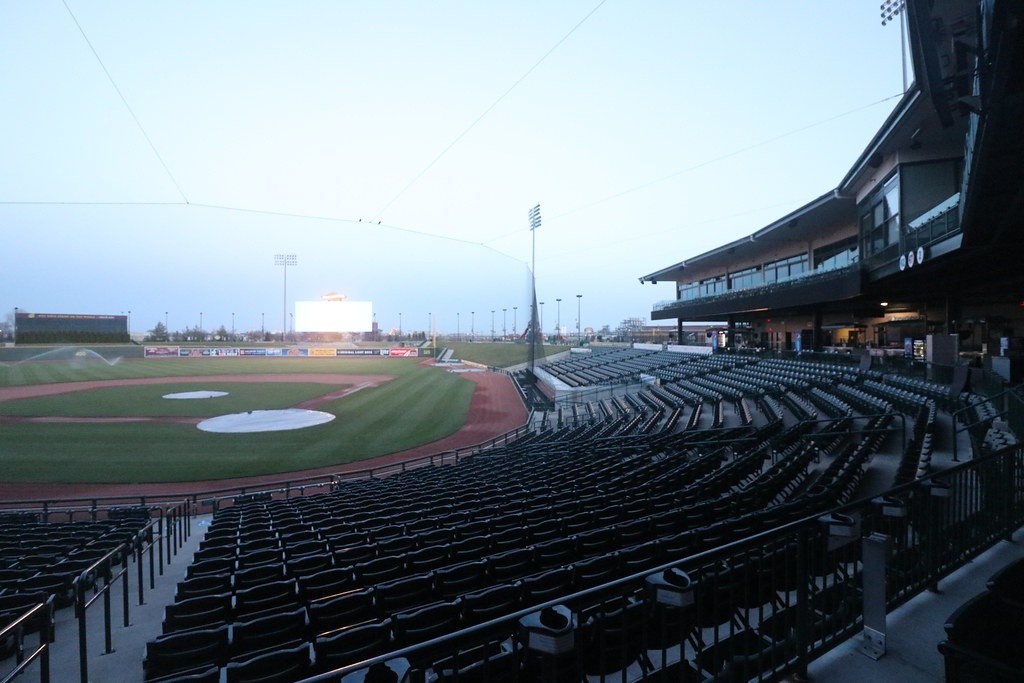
[457,313,460,341]
[429,313,431,340]
[399,312,401,337]
[556,299,562,345]
[576,295,582,348]
[540,302,544,345]
[472,312,474,341]
[274,254,297,344]
[503,309,507,343]
[166,312,168,332]
[290,312,293,338]
[232,313,235,343]
[527,203,542,409]
[200,312,203,333]
[262,313,265,339]
[513,307,517,341]
[491,311,495,342]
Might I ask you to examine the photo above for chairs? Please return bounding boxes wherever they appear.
[0,344,1024,683]
